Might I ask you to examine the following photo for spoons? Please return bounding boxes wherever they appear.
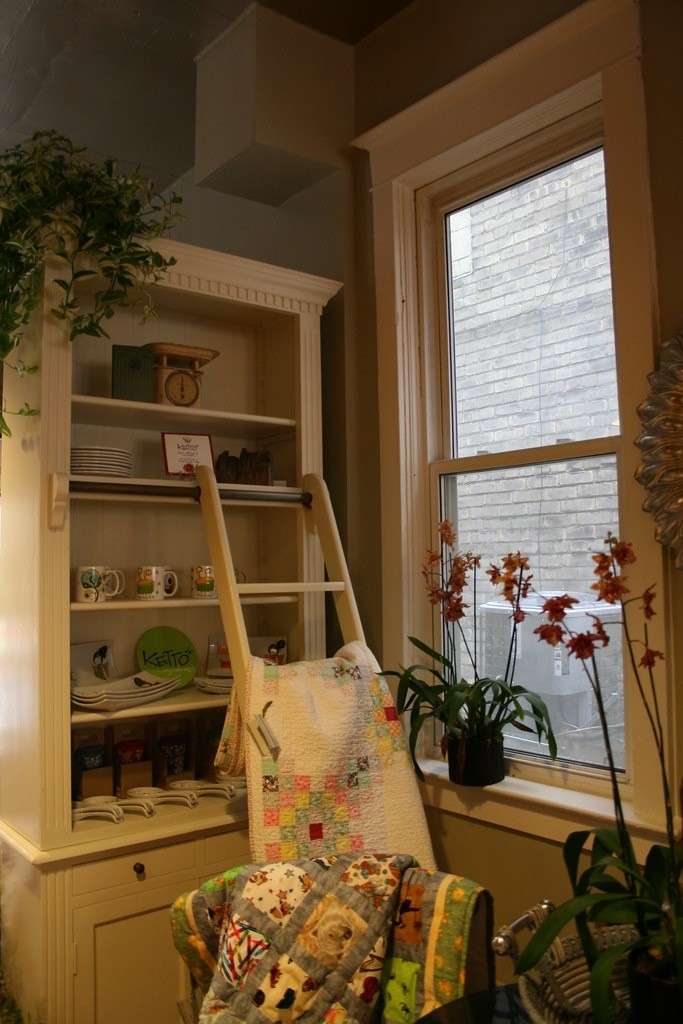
[72,776,246,823]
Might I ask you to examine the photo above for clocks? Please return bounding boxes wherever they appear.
[112,341,221,409]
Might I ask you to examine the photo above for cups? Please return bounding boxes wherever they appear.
[191,565,217,599]
[76,565,119,603]
[136,565,179,601]
[105,567,125,599]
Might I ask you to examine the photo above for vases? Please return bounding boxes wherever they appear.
[448,733,506,787]
[628,944,682,1023]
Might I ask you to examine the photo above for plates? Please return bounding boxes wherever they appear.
[71,446,133,478]
[71,670,182,712]
[70,640,119,685]
[136,625,198,690]
[193,677,234,693]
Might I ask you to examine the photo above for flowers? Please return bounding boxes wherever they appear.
[375,519,558,783]
[514,531,683,1024]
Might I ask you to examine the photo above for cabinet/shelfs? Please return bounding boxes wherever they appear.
[0,222,345,1024]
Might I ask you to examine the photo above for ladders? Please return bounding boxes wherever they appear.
[194,463,367,721]
